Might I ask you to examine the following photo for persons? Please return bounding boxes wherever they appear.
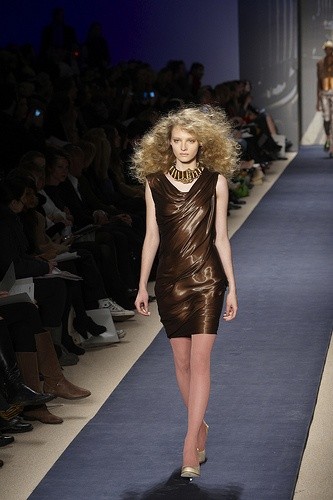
[130,103,241,481]
[0,10,292,448]
[315,39,333,158]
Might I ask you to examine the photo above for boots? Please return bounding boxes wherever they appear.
[34,330,91,399]
[1,350,55,406]
[1,406,34,432]
[15,352,63,424]
[48,328,80,365]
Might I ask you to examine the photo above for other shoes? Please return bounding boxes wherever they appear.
[0,434,14,447]
[229,200,246,209]
[324,140,330,153]
[329,152,333,159]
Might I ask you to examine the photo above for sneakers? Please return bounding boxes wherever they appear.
[97,298,136,321]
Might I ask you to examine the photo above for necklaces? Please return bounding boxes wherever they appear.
[166,161,206,184]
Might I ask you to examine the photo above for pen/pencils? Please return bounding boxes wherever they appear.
[38,256,49,262]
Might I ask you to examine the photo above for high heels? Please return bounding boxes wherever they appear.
[180,451,200,482]
[74,316,107,339]
[197,425,210,462]
[62,335,85,356]
